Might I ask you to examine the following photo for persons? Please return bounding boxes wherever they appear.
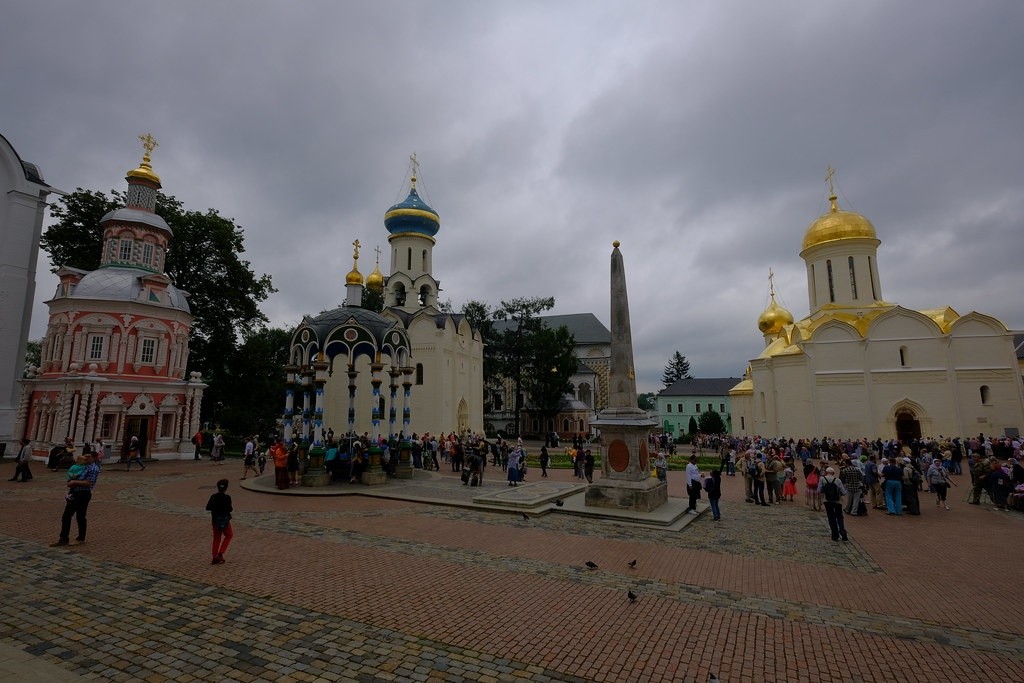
[18,439,32,482]
[194,427,203,460]
[82,443,91,455]
[8,439,33,481]
[125,433,145,472]
[205,479,234,564]
[817,466,848,541]
[94,438,105,471]
[64,455,88,500]
[65,437,73,450]
[51,437,76,472]
[49,451,100,548]
[211,426,1024,520]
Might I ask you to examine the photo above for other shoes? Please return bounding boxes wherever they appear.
[688,509,700,515]
[761,504,770,506]
[712,516,720,521]
[212,556,225,564]
[69,537,85,545]
[775,502,779,505]
[49,540,68,547]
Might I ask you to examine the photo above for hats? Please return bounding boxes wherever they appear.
[826,467,835,474]
[903,458,912,462]
[584,450,591,453]
[658,453,665,460]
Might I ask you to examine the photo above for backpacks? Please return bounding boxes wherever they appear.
[749,461,762,480]
[823,476,841,501]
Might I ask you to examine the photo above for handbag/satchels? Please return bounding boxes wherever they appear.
[461,474,467,483]
[806,466,819,485]
[945,482,952,488]
[471,475,478,486]
[790,476,797,484]
